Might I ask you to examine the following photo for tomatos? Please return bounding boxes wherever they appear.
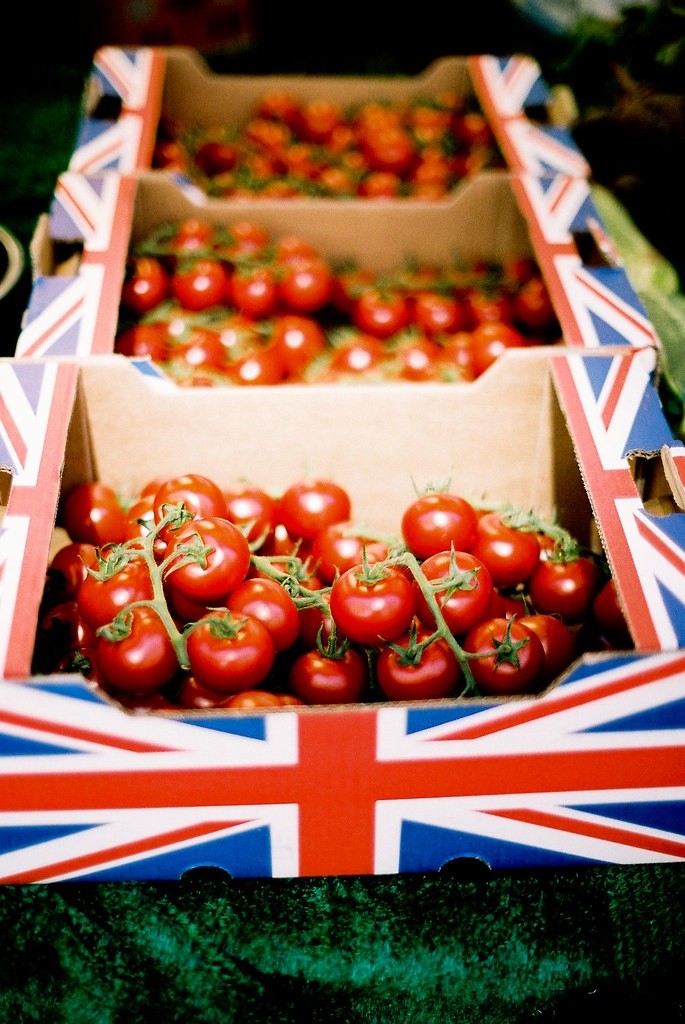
[104,218,556,391]
[34,472,630,710]
[152,84,499,201]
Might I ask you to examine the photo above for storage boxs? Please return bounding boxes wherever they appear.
[0,46,684,888]
